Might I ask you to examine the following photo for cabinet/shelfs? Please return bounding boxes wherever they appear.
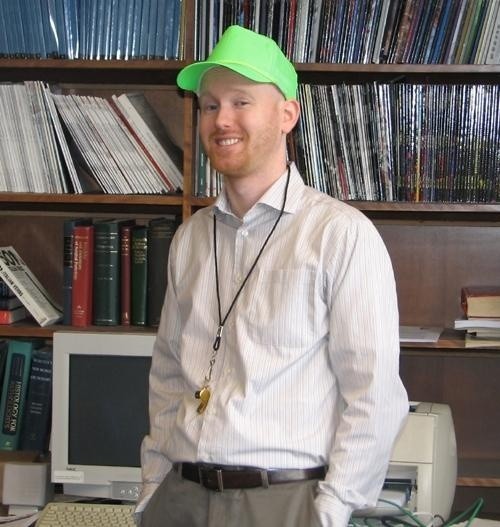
[0,0,500,521]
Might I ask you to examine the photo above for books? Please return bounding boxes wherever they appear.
[454,287,499,348]
[19,345,53,453]
[1,244,64,328]
[65,218,175,332]
[194,79,498,205]
[2,80,183,195]
[1,340,38,453]
[196,2,499,65]
[1,1,185,61]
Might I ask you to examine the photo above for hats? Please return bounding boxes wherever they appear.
[174,24,300,105]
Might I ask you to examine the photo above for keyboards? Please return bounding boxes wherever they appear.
[35,502,139,527]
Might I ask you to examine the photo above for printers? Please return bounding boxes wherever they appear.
[353,401,460,527]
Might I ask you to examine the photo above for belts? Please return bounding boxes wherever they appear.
[174,462,327,490]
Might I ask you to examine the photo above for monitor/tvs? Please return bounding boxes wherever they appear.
[47,330,156,504]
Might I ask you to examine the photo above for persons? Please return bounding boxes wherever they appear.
[128,23,411,527]
